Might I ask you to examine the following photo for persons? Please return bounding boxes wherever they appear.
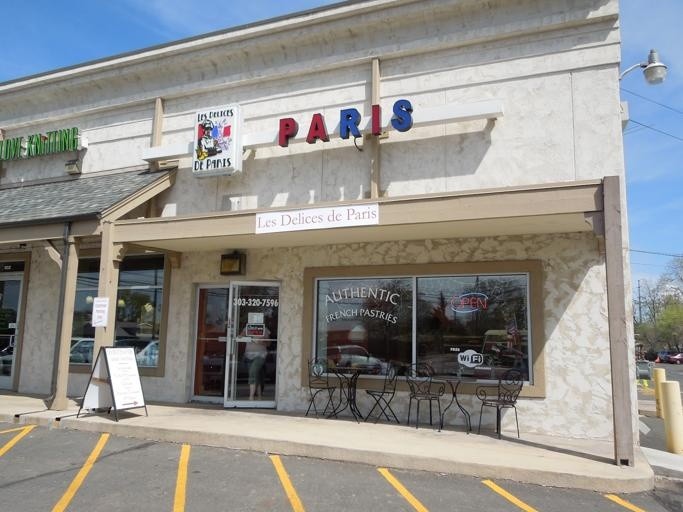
[237,322,271,401]
[198,118,220,157]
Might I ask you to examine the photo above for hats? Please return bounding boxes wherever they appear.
[202,119,214,129]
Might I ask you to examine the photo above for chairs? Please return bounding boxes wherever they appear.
[304,355,525,440]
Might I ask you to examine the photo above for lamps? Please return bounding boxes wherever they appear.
[617,49,669,85]
[64,160,82,175]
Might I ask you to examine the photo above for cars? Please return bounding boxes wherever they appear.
[70,338,159,366]
[659,350,683,365]
[322,345,395,376]
